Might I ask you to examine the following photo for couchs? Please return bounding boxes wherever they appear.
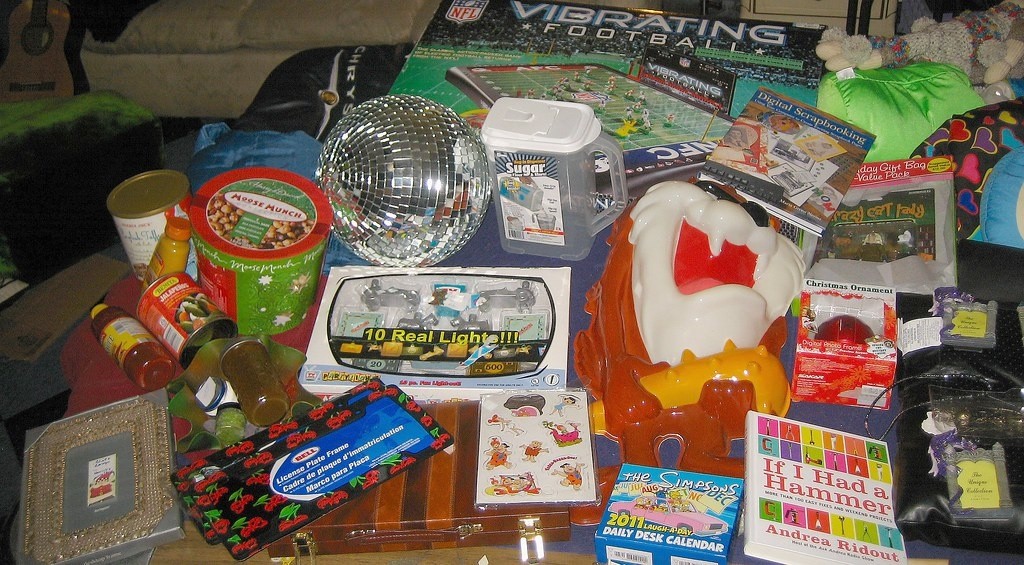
[60,0,441,118]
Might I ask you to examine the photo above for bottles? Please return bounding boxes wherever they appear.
[140,217,192,297]
[214,402,247,446]
[89,303,175,392]
[195,376,239,419]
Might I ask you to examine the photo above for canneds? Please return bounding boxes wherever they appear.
[106,169,198,287]
[136,271,238,372]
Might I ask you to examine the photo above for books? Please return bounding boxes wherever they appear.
[744,411,908,565]
[697,87,877,238]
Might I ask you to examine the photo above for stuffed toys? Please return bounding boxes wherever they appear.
[816,0,1024,249]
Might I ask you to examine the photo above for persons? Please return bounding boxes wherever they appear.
[527,67,674,130]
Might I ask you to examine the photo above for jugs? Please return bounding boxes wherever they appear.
[481,97,627,261]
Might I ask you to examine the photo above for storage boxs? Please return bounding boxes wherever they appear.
[789,155,956,318]
[790,280,899,408]
[13,392,185,565]
[593,463,744,564]
[297,266,570,401]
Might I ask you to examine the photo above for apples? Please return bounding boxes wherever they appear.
[813,315,876,345]
